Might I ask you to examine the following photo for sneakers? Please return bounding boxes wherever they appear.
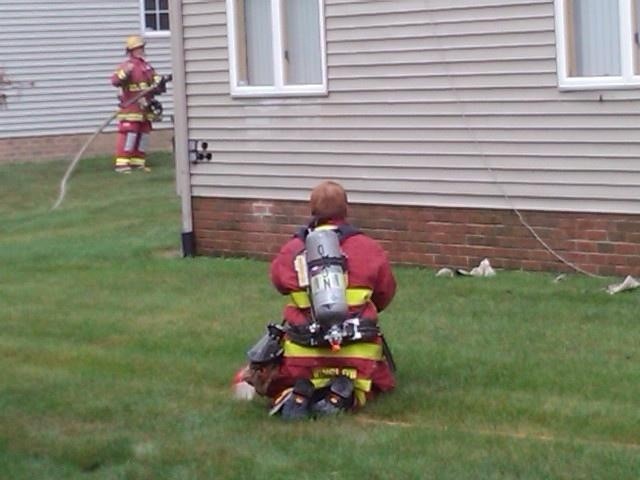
[280,377,315,421]
[312,376,355,418]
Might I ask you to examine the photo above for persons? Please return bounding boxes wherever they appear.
[109,35,168,176]
[232,179,401,419]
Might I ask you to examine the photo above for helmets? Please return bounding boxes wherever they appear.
[125,35,147,51]
